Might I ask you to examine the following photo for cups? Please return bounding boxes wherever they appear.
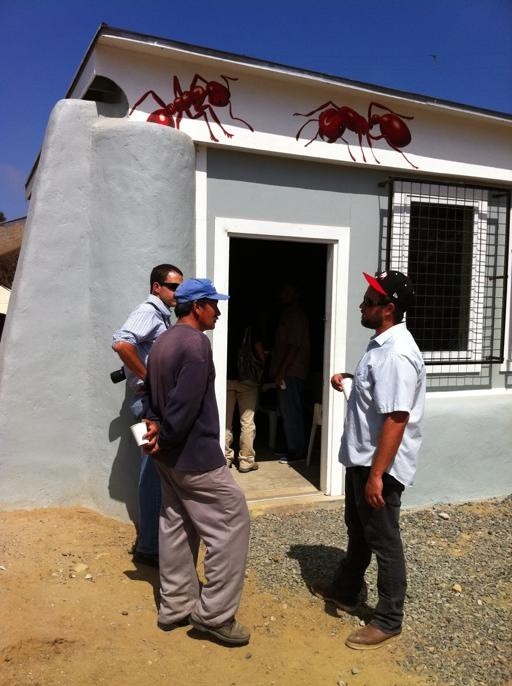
[280,380,286,389]
[341,377,352,401]
[130,421,149,445]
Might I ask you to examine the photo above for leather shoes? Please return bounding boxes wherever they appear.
[310,580,360,614]
[345,622,401,650]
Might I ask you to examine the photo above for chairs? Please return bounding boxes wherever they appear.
[256,403,278,449]
[306,402,323,466]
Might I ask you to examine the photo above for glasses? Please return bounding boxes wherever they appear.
[160,282,179,291]
[364,296,386,307]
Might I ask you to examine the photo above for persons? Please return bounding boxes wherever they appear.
[229,280,311,472]
[309,270,427,650]
[111,263,184,566]
[141,277,251,644]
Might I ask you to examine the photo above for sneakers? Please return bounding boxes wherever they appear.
[133,551,159,568]
[188,612,251,645]
[239,447,308,473]
[157,616,189,632]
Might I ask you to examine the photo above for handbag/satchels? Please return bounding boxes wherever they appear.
[228,351,265,386]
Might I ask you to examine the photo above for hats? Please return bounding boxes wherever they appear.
[363,271,415,310]
[173,277,228,304]
